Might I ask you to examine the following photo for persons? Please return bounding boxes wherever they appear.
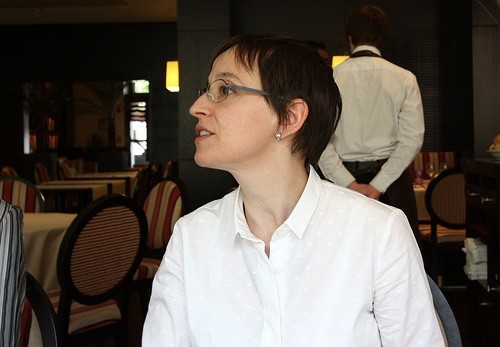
[303,39,331,66]
[141,32,450,347]
[319,6,426,243]
[0,198,26,347]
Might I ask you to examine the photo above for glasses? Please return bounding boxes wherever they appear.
[197,79,291,104]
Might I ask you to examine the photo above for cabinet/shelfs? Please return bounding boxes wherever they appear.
[461,151,500,307]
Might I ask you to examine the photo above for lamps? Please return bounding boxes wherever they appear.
[332,55,350,70]
[165,61,180,92]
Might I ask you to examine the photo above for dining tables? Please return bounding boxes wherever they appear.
[22,213,78,293]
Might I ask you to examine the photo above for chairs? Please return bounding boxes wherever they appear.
[30,157,146,213]
[20,271,61,347]
[424,168,466,287]
[409,152,455,222]
[0,176,46,214]
[46,194,148,346]
[119,176,188,319]
